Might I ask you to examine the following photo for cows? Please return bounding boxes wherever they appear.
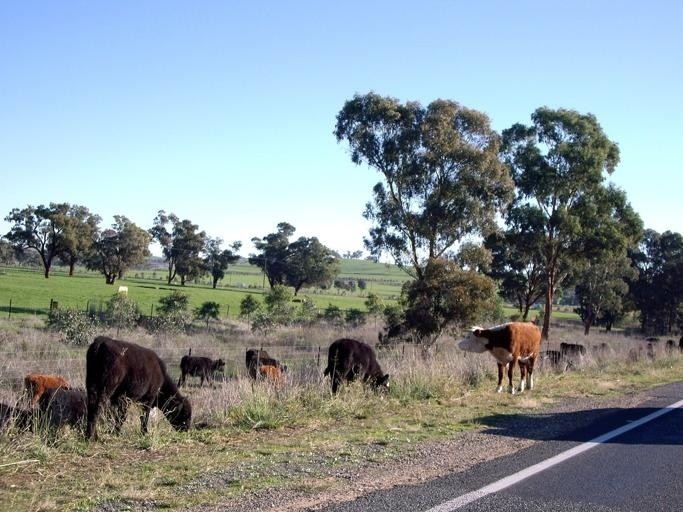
[175,350,227,391]
[79,335,194,444]
[1,373,88,438]
[560,342,587,357]
[321,336,392,399]
[456,320,545,393]
[540,350,565,371]
[245,348,288,391]
